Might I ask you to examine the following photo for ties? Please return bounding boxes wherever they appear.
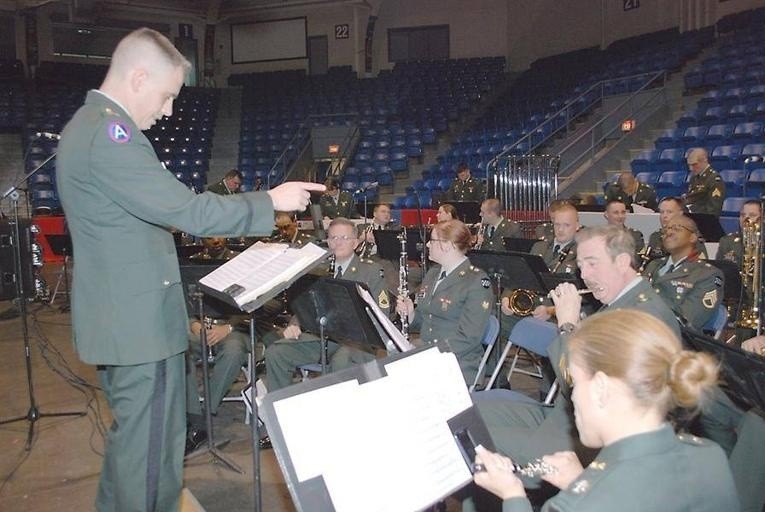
[552,245,561,256]
[663,264,674,275]
[334,266,343,281]
[489,226,495,238]
[436,271,446,282]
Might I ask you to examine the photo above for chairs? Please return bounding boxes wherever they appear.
[720,168,749,196]
[658,148,684,177]
[613,173,620,181]
[465,145,476,160]
[437,154,445,161]
[390,149,408,172]
[354,186,378,204]
[0,61,224,214]
[297,364,323,381]
[237,166,256,178]
[344,166,358,180]
[448,161,460,179]
[351,149,370,166]
[489,144,501,160]
[704,304,729,338]
[391,195,406,209]
[407,140,421,156]
[501,144,513,156]
[468,160,478,176]
[685,147,712,172]
[377,166,393,185]
[505,346,544,381]
[742,144,765,168]
[423,177,436,190]
[447,163,459,178]
[477,160,487,178]
[468,314,499,393]
[630,149,660,177]
[723,198,758,216]
[445,148,453,162]
[255,165,270,177]
[412,180,423,191]
[440,164,451,178]
[437,177,452,192]
[438,162,448,177]
[431,163,438,178]
[454,146,464,161]
[404,193,418,208]
[712,145,742,170]
[422,170,433,181]
[419,192,432,209]
[476,145,488,161]
[473,317,559,405]
[237,150,256,166]
[256,150,274,166]
[360,166,376,185]
[517,138,529,151]
[197,351,250,423]
[635,172,659,185]
[240,177,252,192]
[342,180,358,191]
[596,195,604,205]
[431,164,439,177]
[227,8,765,148]
[746,168,765,197]
[371,149,390,167]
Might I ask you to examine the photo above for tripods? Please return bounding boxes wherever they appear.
[179,298,246,475]
[249,313,261,512]
[1,149,88,450]
[315,315,329,376]
[58,249,72,313]
[493,273,503,388]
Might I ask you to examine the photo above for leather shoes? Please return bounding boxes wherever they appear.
[184,426,208,455]
[257,434,273,449]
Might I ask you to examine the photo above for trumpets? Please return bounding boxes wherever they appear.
[734,197,764,332]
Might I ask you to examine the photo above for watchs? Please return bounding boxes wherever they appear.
[559,322,576,334]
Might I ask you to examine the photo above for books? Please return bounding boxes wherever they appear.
[199,239,327,308]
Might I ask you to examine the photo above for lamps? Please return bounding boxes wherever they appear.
[621,120,635,132]
[328,145,340,154]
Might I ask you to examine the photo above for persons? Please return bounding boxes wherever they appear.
[528,206,584,274]
[473,309,741,512]
[610,170,658,214]
[329,219,493,390]
[208,170,246,196]
[535,200,572,240]
[444,163,485,203]
[604,200,645,268]
[698,330,765,511]
[186,236,246,455]
[644,215,723,329]
[257,218,390,451]
[716,199,765,266]
[354,204,404,261]
[319,177,360,220]
[642,196,708,271]
[263,211,315,247]
[467,199,521,251]
[675,147,726,215]
[428,204,459,225]
[54,25,331,512]
[469,223,683,489]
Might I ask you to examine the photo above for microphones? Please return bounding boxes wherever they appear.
[37,132,61,140]
[356,181,379,193]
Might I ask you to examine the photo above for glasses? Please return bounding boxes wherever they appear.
[328,235,357,241]
[660,223,695,233]
[429,238,451,246]
